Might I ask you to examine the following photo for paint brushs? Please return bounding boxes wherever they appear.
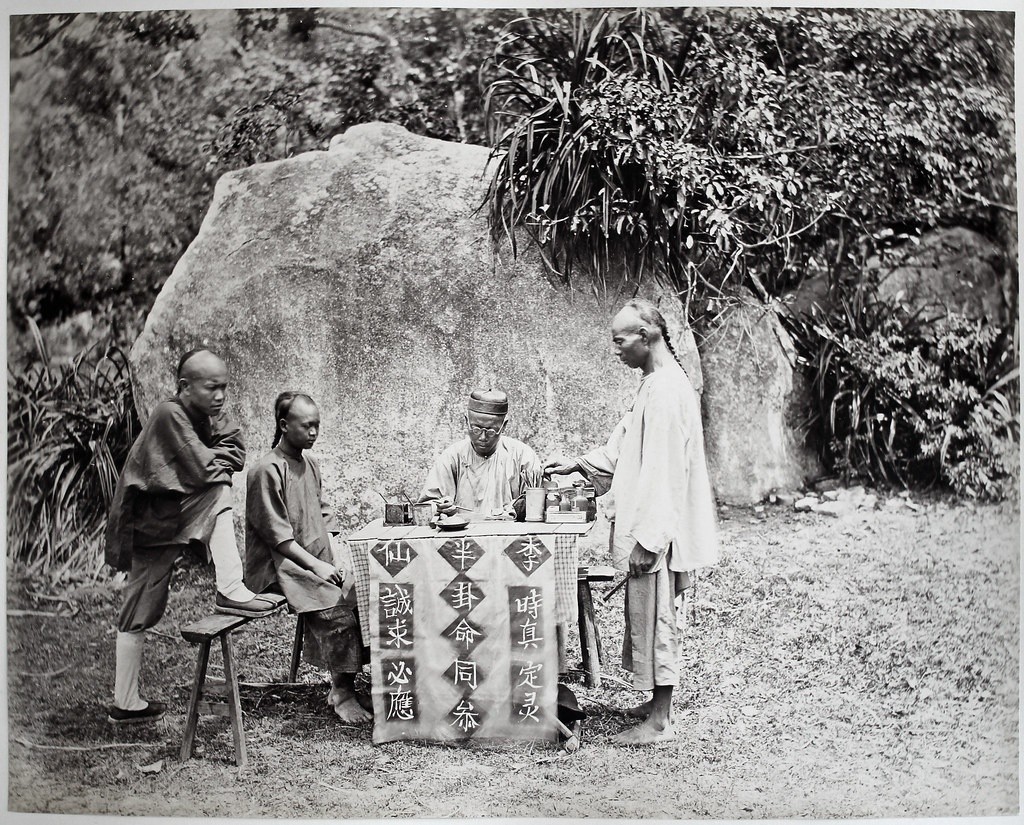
[371,488,389,503]
[520,466,548,489]
[401,490,415,506]
[431,500,474,513]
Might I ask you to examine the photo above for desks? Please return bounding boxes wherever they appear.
[344,514,597,752]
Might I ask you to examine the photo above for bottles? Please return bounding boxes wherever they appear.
[572,488,588,511]
[560,495,570,511]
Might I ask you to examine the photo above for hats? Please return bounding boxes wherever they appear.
[558,684,586,720]
[469,389,508,414]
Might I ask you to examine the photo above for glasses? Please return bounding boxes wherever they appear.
[467,416,504,437]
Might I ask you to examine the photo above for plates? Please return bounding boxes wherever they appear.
[436,519,468,528]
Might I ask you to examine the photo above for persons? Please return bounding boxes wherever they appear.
[541,297,718,746]
[416,388,541,522]
[245,392,373,724]
[104,348,277,722]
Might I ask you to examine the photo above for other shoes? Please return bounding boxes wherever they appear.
[109,699,167,723]
[214,590,278,617]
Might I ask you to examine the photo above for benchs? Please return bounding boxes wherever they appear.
[574,566,615,689]
[182,592,303,766]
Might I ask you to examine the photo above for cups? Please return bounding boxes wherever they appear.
[525,488,545,521]
[414,503,433,526]
